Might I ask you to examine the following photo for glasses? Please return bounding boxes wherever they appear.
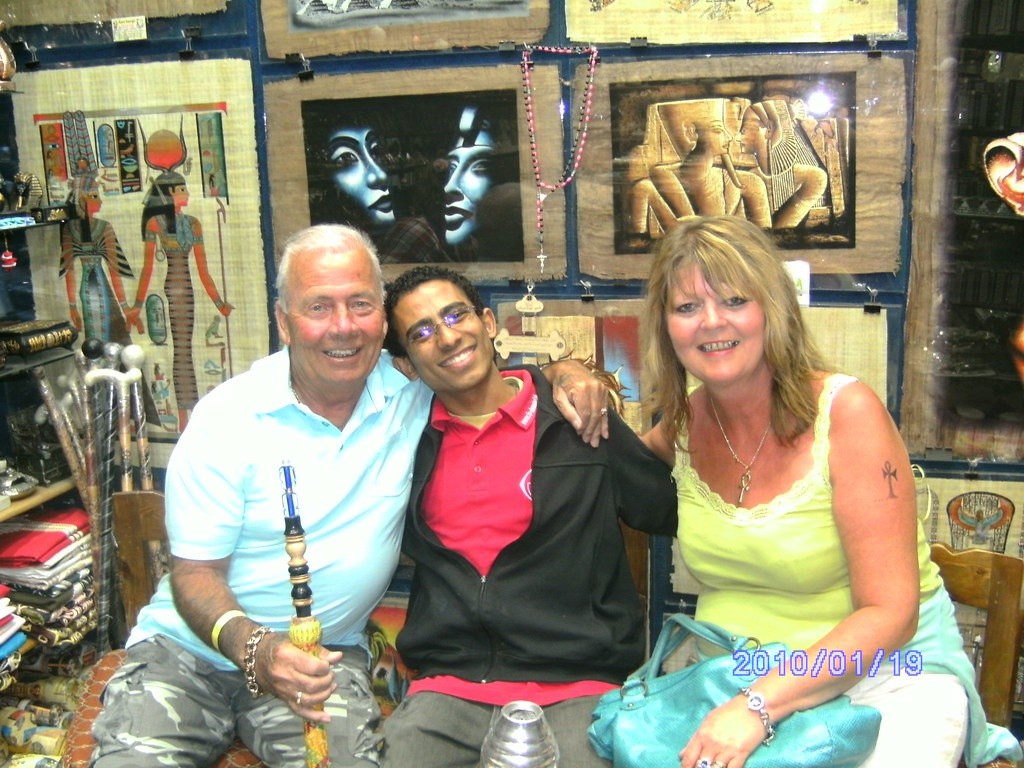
[401,305,477,353]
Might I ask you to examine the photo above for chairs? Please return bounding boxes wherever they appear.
[62,489,265,768]
[928,543,1024,768]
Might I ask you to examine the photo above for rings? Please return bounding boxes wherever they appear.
[601,408,607,414]
[296,691,302,705]
[711,760,727,768]
[696,758,711,768]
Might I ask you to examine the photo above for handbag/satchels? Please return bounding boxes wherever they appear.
[586,613,883,768]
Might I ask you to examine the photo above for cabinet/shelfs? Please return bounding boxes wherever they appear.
[0,89,75,523]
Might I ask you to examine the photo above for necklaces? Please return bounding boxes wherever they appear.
[520,44,598,273]
[707,388,772,503]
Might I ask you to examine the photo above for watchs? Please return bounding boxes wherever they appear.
[739,687,777,747]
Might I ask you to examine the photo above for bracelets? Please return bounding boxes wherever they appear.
[243,627,273,699]
[211,609,247,654]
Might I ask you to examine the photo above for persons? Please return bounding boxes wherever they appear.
[87,224,625,768]
[637,214,1024,768]
[380,268,679,768]
[627,98,828,240]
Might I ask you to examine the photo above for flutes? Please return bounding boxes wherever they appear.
[279,461,329,768]
